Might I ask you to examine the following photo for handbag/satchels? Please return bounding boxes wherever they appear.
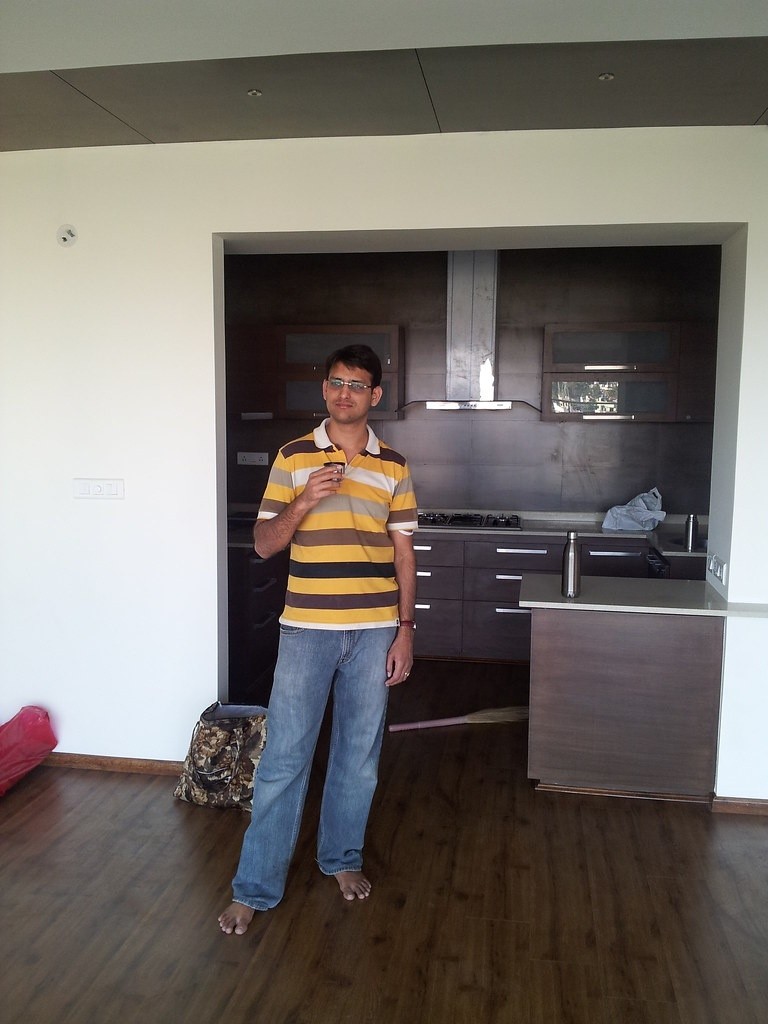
[172,701,268,813]
[601,487,665,530]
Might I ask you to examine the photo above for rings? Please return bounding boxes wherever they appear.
[404,672,410,676]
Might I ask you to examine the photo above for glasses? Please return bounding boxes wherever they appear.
[326,378,376,392]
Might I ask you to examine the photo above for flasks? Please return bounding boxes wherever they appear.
[561,530,581,598]
[684,514,699,551]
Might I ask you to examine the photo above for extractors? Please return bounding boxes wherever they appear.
[425,401,514,411]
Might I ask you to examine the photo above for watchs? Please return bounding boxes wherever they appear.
[400,620,417,632]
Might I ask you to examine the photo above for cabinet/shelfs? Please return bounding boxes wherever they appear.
[225,323,406,422]
[411,532,570,665]
[228,547,290,673]
[540,323,718,423]
[577,536,650,578]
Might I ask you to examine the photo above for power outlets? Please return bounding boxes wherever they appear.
[237,452,270,466]
[707,551,726,585]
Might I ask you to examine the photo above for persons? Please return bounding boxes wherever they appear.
[217,344,419,934]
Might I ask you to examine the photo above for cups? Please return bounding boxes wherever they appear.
[323,461,346,482]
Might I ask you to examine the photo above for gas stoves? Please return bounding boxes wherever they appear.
[414,511,523,531]
